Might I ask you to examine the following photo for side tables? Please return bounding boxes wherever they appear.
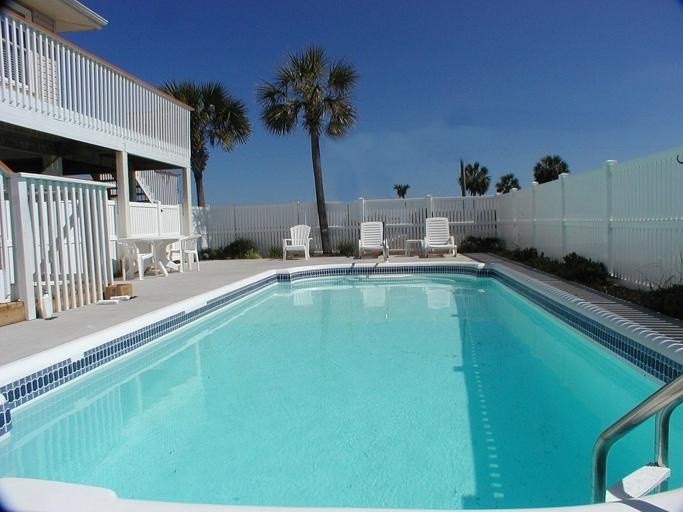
[404,239,423,257]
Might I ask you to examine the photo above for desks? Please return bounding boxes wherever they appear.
[117,235,179,279]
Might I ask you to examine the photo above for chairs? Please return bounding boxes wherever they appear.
[423,217,458,258]
[110,238,152,281]
[357,221,388,258]
[281,224,312,261]
[169,233,203,272]
